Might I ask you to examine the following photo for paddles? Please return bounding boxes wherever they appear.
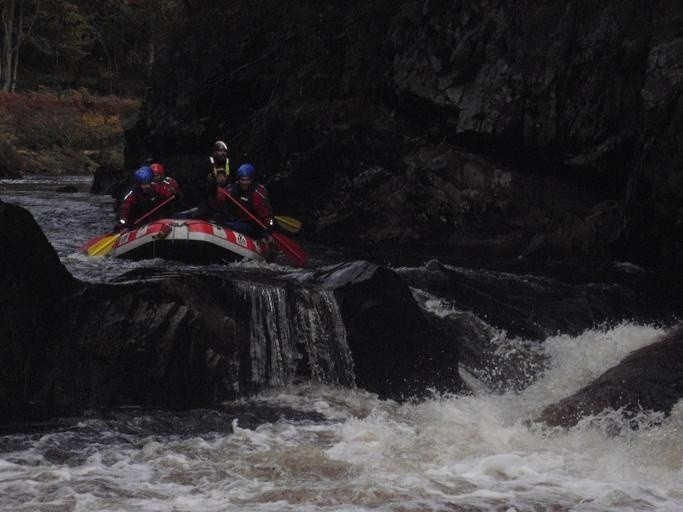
[221,189,308,266]
[273,216,301,233]
[81,195,177,256]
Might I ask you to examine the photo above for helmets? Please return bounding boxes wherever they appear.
[135,167,154,183]
[238,164,254,177]
[213,140,229,152]
[151,163,164,174]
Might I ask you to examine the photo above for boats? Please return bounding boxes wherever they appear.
[107,217,272,265]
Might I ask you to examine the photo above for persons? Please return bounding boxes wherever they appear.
[208,163,279,237]
[202,140,235,217]
[118,166,183,234]
[149,163,182,220]
